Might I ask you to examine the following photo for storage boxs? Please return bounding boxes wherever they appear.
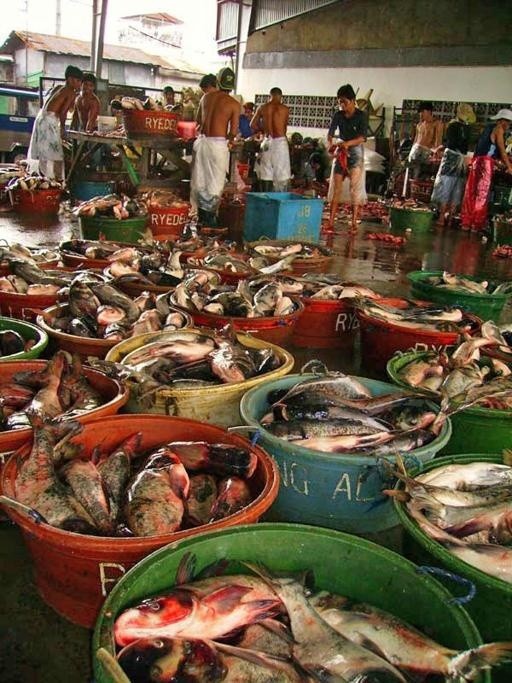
[242,189,325,245]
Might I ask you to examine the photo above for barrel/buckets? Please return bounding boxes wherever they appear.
[0,163,25,213]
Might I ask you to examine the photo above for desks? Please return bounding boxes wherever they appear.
[64,128,193,190]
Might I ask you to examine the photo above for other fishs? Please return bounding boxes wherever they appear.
[7,413,275,532]
[87,550,508,683]
[0,224,482,422]
[245,368,452,458]
[378,441,512,591]
[392,319,511,415]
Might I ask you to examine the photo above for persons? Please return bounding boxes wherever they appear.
[308,152,325,182]
[250,88,294,190]
[321,83,368,231]
[190,67,240,220]
[70,74,98,133]
[453,232,484,276]
[239,103,254,164]
[326,231,356,260]
[163,86,174,104]
[459,108,512,230]
[30,65,84,181]
[402,102,444,197]
[430,103,477,226]
[432,227,452,271]
[198,72,218,94]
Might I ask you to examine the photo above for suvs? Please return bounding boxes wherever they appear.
[0,82,76,161]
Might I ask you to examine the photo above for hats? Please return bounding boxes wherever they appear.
[216,67,236,91]
[490,108,512,122]
[456,103,478,124]
